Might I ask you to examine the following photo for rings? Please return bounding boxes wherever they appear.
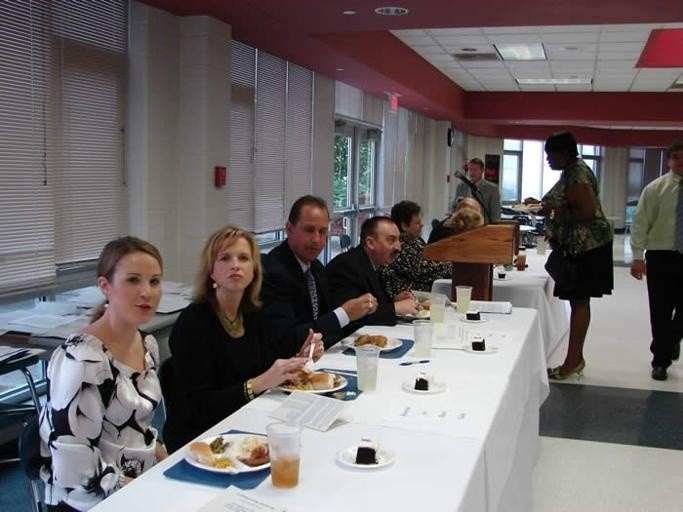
[637,274,641,279]
[416,300,420,308]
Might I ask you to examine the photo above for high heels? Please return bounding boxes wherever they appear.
[547,359,584,380]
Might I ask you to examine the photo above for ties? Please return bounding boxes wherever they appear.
[674,179,683,254]
[305,267,319,319]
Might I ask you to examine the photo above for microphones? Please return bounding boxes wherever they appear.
[455,170,476,193]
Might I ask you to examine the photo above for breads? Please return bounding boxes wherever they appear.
[236,435,270,467]
[310,372,335,391]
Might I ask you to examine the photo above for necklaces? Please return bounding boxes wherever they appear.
[216,306,246,335]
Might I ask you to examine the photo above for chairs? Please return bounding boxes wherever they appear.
[16,415,47,511]
[156,355,194,456]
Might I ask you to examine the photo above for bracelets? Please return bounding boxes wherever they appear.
[244,377,255,400]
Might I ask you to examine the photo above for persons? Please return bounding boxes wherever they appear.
[262,194,379,367]
[382,200,452,303]
[326,214,426,346]
[453,156,501,222]
[39,235,171,512]
[426,208,484,245]
[453,197,480,212]
[629,139,683,382]
[162,225,328,459]
[522,128,615,380]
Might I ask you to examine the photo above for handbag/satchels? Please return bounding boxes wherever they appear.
[544,207,581,295]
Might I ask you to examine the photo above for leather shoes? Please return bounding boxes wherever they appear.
[670,341,679,360]
[652,364,667,381]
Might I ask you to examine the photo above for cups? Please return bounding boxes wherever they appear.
[516,254,526,270]
[535,234,546,255]
[267,421,302,490]
[454,285,473,314]
[412,319,433,357]
[428,293,446,322]
[502,262,512,271]
[353,344,382,393]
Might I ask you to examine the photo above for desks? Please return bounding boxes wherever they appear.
[0,279,193,377]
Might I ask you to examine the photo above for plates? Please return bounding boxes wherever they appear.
[493,273,513,281]
[337,445,394,469]
[183,432,270,475]
[273,308,429,393]
[403,317,494,394]
[508,206,541,212]
